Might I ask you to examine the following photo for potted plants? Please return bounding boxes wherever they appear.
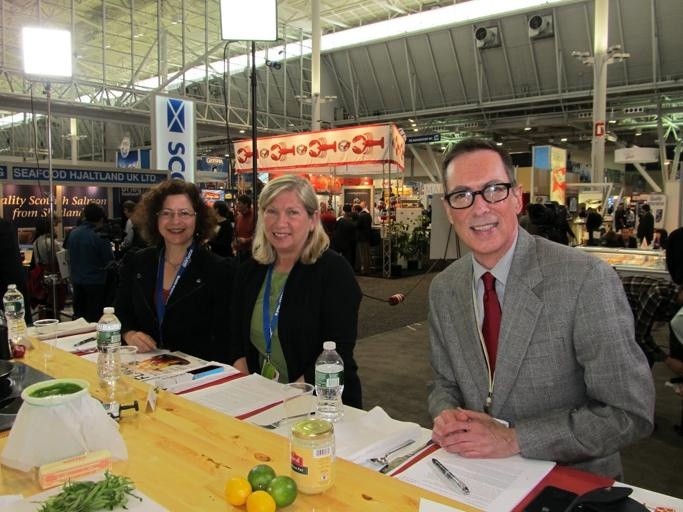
[387,214,432,272]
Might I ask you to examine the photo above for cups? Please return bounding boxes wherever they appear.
[32,319,58,354]
[105,345,138,394]
[281,382,314,430]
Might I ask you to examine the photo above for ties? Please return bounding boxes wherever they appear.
[476,272,505,375]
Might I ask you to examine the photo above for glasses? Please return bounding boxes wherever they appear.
[158,207,198,219]
[442,183,514,209]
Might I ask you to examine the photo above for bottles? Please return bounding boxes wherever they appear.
[291,420,336,494]
[96,307,121,382]
[0,309,10,359]
[3,285,27,343]
[315,341,344,425]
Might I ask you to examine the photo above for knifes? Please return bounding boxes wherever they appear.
[378,438,433,474]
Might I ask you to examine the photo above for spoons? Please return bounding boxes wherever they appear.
[365,439,415,467]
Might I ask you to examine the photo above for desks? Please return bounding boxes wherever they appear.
[1,324,683,511]
[574,246,671,282]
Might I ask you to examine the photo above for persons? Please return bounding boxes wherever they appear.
[116,200,138,254]
[232,194,258,257]
[664,227,681,385]
[616,274,683,378]
[576,206,588,219]
[615,201,627,229]
[64,200,115,321]
[208,199,236,258]
[614,229,637,249]
[319,197,386,276]
[220,173,361,400]
[623,204,636,227]
[634,203,656,248]
[653,226,669,250]
[107,179,219,362]
[427,137,656,486]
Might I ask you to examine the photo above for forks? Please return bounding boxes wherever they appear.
[251,411,315,429]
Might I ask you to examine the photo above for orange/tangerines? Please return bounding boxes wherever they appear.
[248,464,276,491]
[266,475,297,507]
[225,478,252,506]
[246,490,276,512]
